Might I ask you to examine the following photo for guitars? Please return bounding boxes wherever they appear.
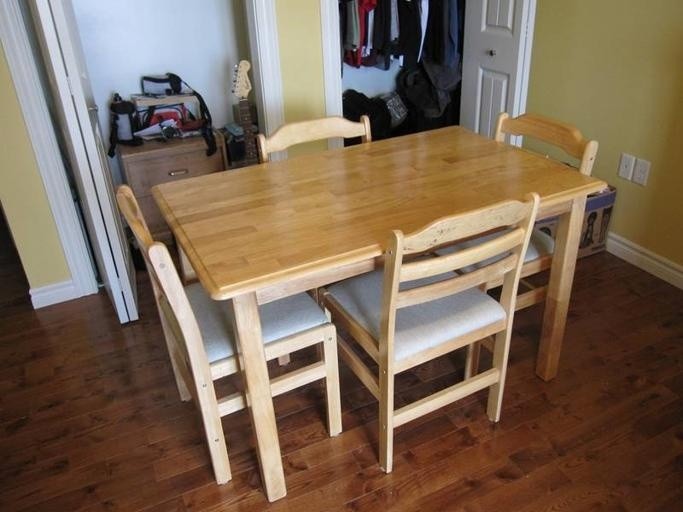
[226,60,261,171]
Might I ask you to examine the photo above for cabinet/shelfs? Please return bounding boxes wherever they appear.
[118,127,228,254]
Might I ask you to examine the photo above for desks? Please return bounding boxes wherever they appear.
[151,126,608,503]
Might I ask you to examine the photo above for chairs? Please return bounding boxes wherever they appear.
[115,183,342,485]
[318,192,539,474]
[429,112,599,385]
[256,114,372,165]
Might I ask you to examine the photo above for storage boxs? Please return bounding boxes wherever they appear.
[533,160,616,262]
